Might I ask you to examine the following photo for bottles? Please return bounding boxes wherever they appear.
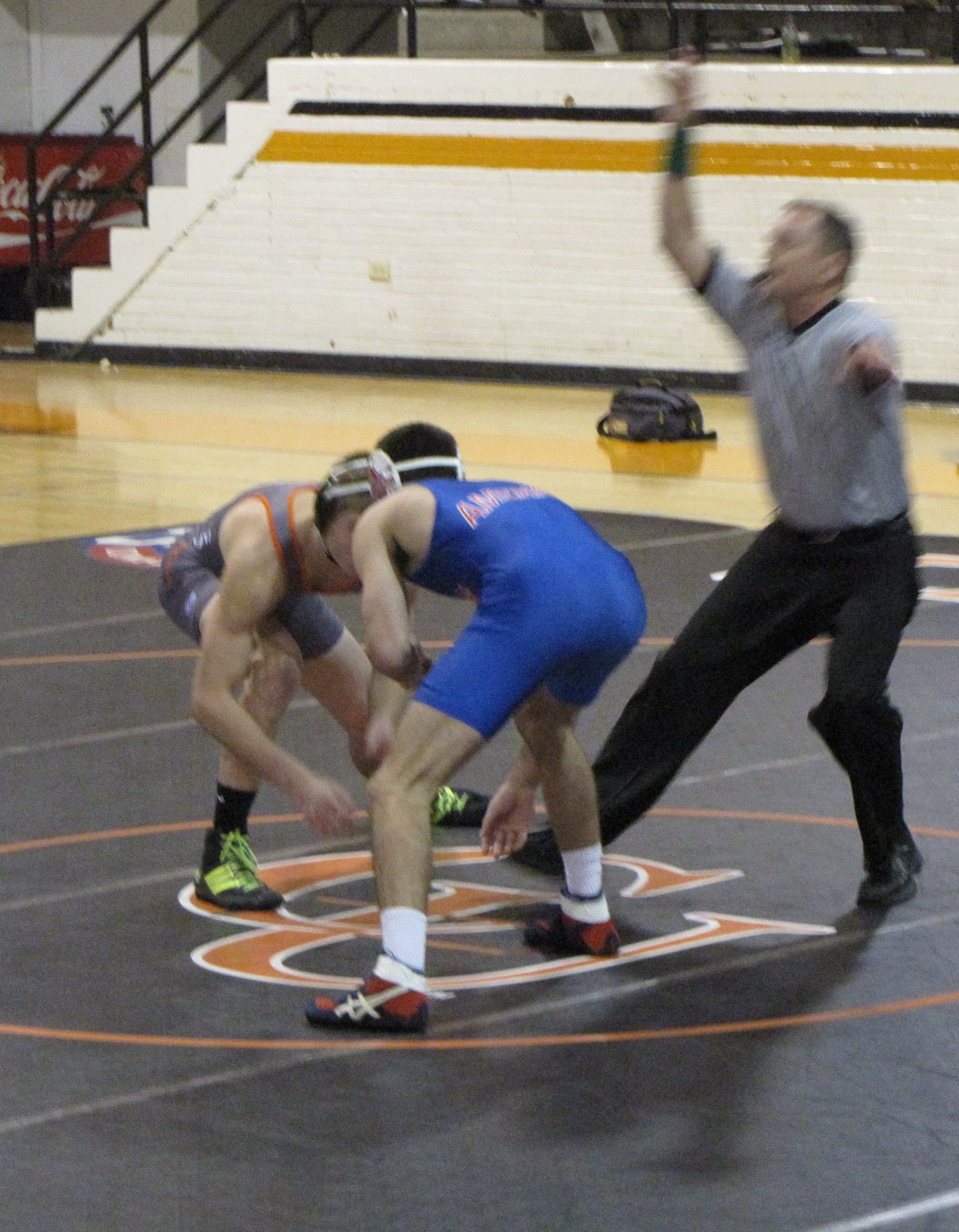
[782,17,799,63]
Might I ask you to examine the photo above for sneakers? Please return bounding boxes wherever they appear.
[509,826,562,876]
[195,824,285,912]
[858,838,924,909]
[304,971,428,1035]
[430,785,490,831]
[522,906,621,958]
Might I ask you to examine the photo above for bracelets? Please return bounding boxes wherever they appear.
[667,124,687,177]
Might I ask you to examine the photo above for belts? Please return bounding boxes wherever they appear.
[796,514,908,546]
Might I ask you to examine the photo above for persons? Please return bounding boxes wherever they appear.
[512,64,926,907]
[305,450,644,1028]
[160,424,492,912]
